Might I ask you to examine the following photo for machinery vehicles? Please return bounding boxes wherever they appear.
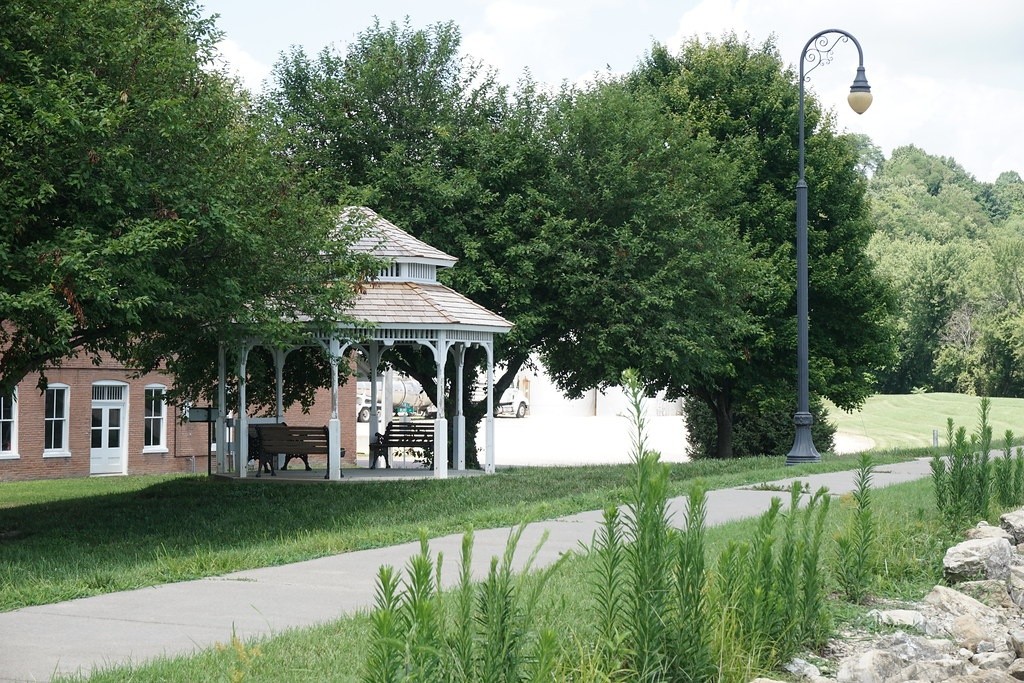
[356,376,437,423]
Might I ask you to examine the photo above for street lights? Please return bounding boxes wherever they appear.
[785,29,873,467]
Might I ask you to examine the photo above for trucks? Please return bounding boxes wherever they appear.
[474,375,530,419]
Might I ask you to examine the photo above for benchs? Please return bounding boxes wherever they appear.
[369,421,434,470]
[247,421,313,473]
[255,424,344,477]
